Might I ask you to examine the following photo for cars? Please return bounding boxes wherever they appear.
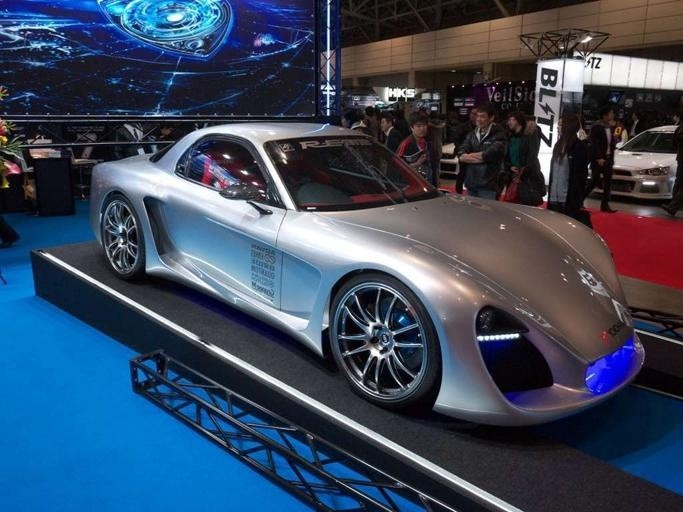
[339,85,378,107]
[439,139,459,175]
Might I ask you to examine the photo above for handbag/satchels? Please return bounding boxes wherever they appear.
[499,168,546,206]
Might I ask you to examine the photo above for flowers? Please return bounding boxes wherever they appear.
[0,85,35,200]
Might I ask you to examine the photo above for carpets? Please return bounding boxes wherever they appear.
[436,184,683,293]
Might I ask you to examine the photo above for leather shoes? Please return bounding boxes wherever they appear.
[600,208,617,212]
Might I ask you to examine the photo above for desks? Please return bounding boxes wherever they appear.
[71,158,105,200]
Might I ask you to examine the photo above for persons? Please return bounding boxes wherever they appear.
[336,103,545,206]
[113,120,155,159]
[0,213,20,249]
[582,107,618,213]
[612,118,628,149]
[548,114,593,228]
[152,123,175,152]
[627,112,643,141]
[662,117,683,218]
[672,112,683,124]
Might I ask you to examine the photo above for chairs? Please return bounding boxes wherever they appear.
[199,143,332,204]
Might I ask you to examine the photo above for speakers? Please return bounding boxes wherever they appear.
[33,156,76,217]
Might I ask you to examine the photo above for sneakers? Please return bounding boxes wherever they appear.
[661,204,675,215]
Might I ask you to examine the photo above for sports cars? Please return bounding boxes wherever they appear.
[88,121,645,427]
